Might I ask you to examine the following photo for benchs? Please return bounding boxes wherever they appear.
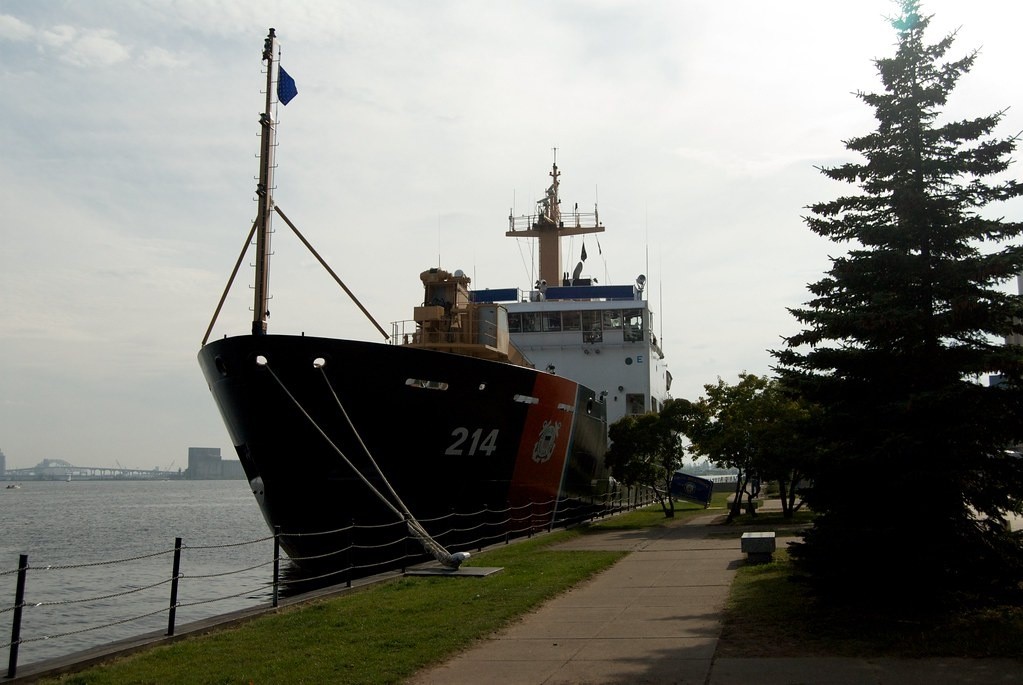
[726,500,763,515]
[742,533,775,562]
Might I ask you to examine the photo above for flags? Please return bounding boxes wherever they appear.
[581,245,587,261]
[278,66,297,106]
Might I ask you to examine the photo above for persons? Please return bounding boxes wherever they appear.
[752,476,758,498]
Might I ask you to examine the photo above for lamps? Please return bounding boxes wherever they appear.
[600,390,609,401]
[637,275,645,289]
[547,363,556,373]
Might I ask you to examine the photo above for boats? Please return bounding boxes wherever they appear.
[198,29,675,593]
